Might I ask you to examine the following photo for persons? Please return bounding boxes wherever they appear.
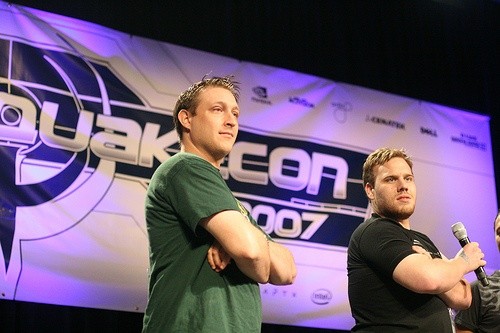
[346,147,486,333]
[454,212,500,333]
[141,71,296,333]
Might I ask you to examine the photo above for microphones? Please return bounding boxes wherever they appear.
[451,222,490,286]
